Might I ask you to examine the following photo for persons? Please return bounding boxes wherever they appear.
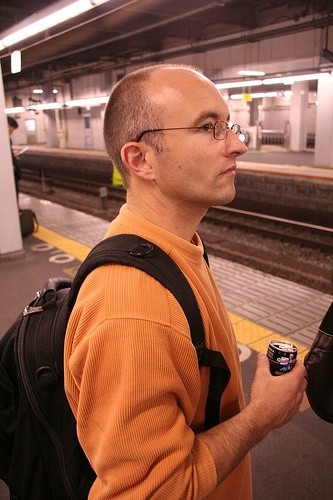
[64,64,308,500]
[6,116,22,210]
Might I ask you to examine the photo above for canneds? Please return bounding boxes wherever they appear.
[267,339,298,376]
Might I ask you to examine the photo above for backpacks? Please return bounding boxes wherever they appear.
[0,234,231,496]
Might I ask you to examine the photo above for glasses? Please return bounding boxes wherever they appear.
[133,121,241,143]
